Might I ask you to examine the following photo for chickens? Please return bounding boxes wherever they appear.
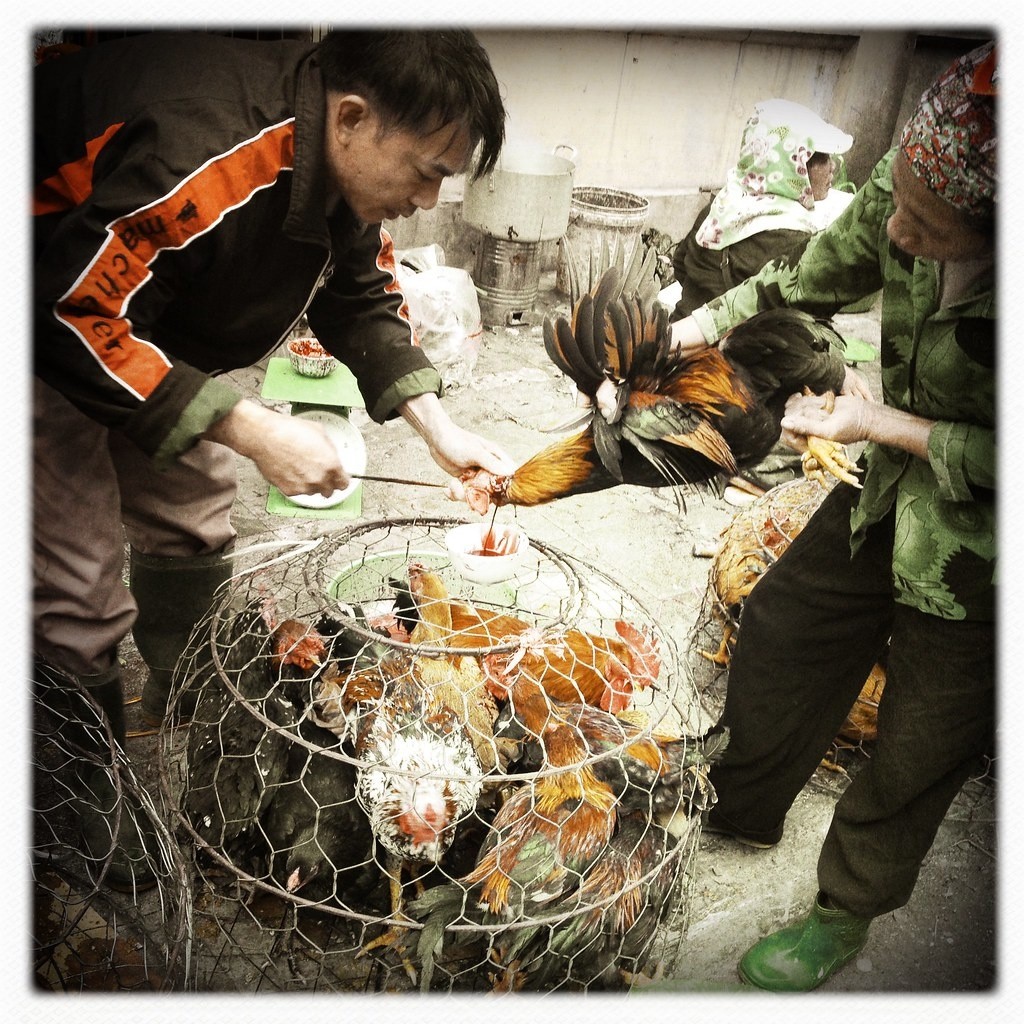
[694,517,889,774]
[457,227,864,517]
[171,565,731,993]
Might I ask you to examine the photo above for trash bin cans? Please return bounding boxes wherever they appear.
[555,186,650,302]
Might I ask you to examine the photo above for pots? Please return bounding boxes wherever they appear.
[463,140,579,245]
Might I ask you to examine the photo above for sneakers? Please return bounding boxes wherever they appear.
[728,467,795,498]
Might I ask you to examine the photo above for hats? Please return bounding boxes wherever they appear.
[753,98,854,156]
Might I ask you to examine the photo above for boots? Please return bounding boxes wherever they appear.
[34,650,163,893]
[700,822,774,849]
[735,890,874,993]
[128,544,236,728]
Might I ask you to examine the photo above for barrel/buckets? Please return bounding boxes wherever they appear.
[555,184,651,302]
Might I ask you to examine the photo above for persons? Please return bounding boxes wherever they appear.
[671,100,878,403]
[670,41,993,990]
[31,28,522,891]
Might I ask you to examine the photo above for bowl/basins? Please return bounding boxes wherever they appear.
[286,336,341,380]
[442,517,534,588]
